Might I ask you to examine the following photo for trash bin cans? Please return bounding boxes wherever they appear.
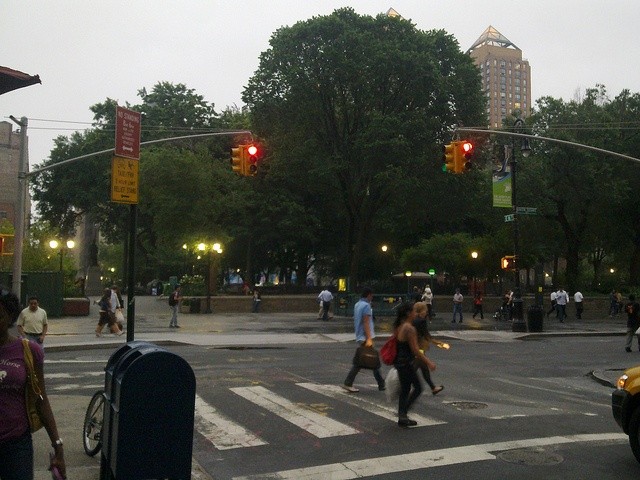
[99,340,196,479]
[527,305,544,332]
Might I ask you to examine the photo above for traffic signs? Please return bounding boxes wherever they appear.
[115,105,141,160]
[516,207,538,216]
[503,214,514,222]
[111,157,139,204]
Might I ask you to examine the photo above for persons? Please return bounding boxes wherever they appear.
[250,288,261,312]
[501,287,519,314]
[414,282,434,306]
[553,286,573,320]
[0,296,64,476]
[100,285,124,336]
[609,287,622,317]
[620,289,639,354]
[571,289,588,320]
[319,287,333,320]
[347,289,385,390]
[17,296,47,350]
[167,286,182,325]
[390,302,431,429]
[451,289,469,323]
[474,290,488,323]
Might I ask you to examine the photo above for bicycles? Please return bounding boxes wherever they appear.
[83,391,105,455]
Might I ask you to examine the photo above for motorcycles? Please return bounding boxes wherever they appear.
[249,274,261,309]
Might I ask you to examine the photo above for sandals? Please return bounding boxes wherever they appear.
[432,386,444,394]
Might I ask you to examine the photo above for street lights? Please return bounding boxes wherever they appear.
[49,240,75,272]
[471,251,478,298]
[197,243,222,313]
[236,269,240,285]
[406,271,412,294]
[183,243,187,276]
[512,119,532,332]
[382,245,387,291]
[429,269,435,290]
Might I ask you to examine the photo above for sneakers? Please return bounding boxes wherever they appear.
[398,420,417,426]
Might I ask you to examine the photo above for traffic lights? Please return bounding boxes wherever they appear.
[244,145,258,176]
[501,256,514,272]
[230,145,244,175]
[456,141,472,173]
[444,142,456,172]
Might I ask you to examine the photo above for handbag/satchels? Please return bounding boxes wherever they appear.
[353,341,380,369]
[22,337,43,433]
[380,335,397,365]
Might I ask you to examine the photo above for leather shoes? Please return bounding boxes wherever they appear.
[345,386,360,392]
